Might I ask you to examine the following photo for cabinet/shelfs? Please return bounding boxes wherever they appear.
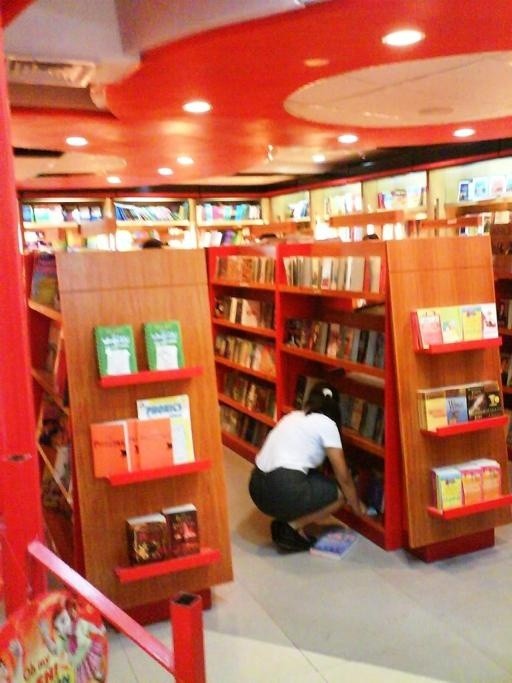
[207,235,512,563]
[25,244,232,630]
[262,147,511,245]
[16,194,271,257]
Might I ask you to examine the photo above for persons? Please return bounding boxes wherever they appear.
[248,380,375,552]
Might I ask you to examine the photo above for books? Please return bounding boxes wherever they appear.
[110,416,143,473]
[220,370,277,423]
[413,295,512,516]
[291,375,384,447]
[89,419,132,479]
[213,295,274,330]
[216,255,275,285]
[160,502,201,555]
[219,405,272,448]
[93,324,140,376]
[125,511,174,566]
[141,318,186,372]
[286,317,383,369]
[282,255,381,293]
[24,253,80,537]
[213,333,276,376]
[317,449,385,514]
[273,156,512,244]
[132,416,175,471]
[24,200,263,251]
[309,530,360,561]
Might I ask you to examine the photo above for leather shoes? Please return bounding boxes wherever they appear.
[271,520,317,552]
